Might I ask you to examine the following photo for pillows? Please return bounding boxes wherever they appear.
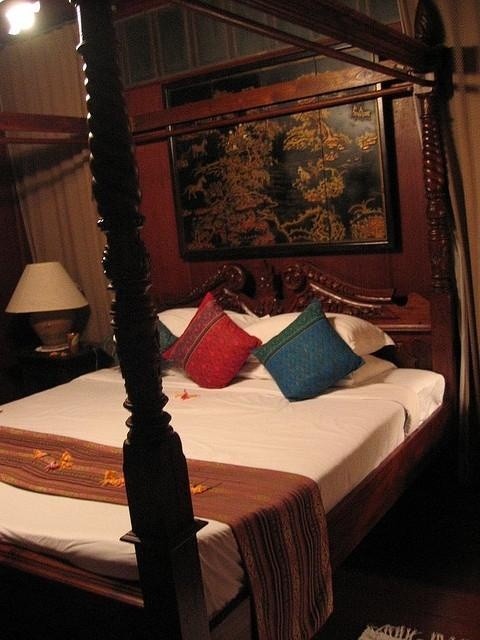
[113,291,396,402]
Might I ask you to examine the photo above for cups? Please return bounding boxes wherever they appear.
[68,333,80,358]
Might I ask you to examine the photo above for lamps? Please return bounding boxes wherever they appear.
[4,262,91,351]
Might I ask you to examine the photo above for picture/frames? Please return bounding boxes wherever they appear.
[159,43,403,261]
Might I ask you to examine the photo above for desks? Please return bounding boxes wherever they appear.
[14,344,102,399]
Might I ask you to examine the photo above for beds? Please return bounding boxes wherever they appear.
[0,260,462,640]
